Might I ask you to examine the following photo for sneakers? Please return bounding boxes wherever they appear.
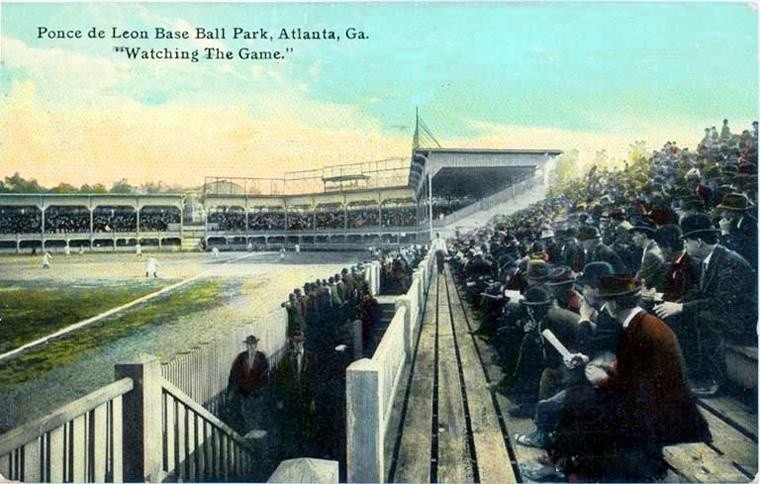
[468,312,571,483]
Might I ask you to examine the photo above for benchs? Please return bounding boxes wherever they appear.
[657,336,759,482]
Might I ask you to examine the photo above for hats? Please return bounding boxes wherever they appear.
[291,331,306,342]
[457,158,758,307]
[243,335,259,343]
